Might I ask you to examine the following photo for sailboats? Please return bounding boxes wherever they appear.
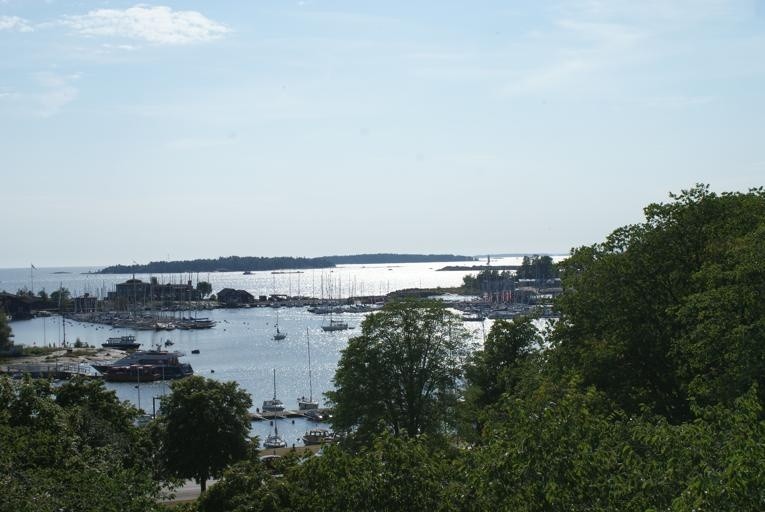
[263,401,287,448]
[273,299,287,340]
[305,265,380,332]
[296,327,319,409]
[456,270,532,323]
[262,369,285,410]
[46,258,218,331]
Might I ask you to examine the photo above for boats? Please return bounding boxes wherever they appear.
[90,344,194,379]
[302,429,334,443]
